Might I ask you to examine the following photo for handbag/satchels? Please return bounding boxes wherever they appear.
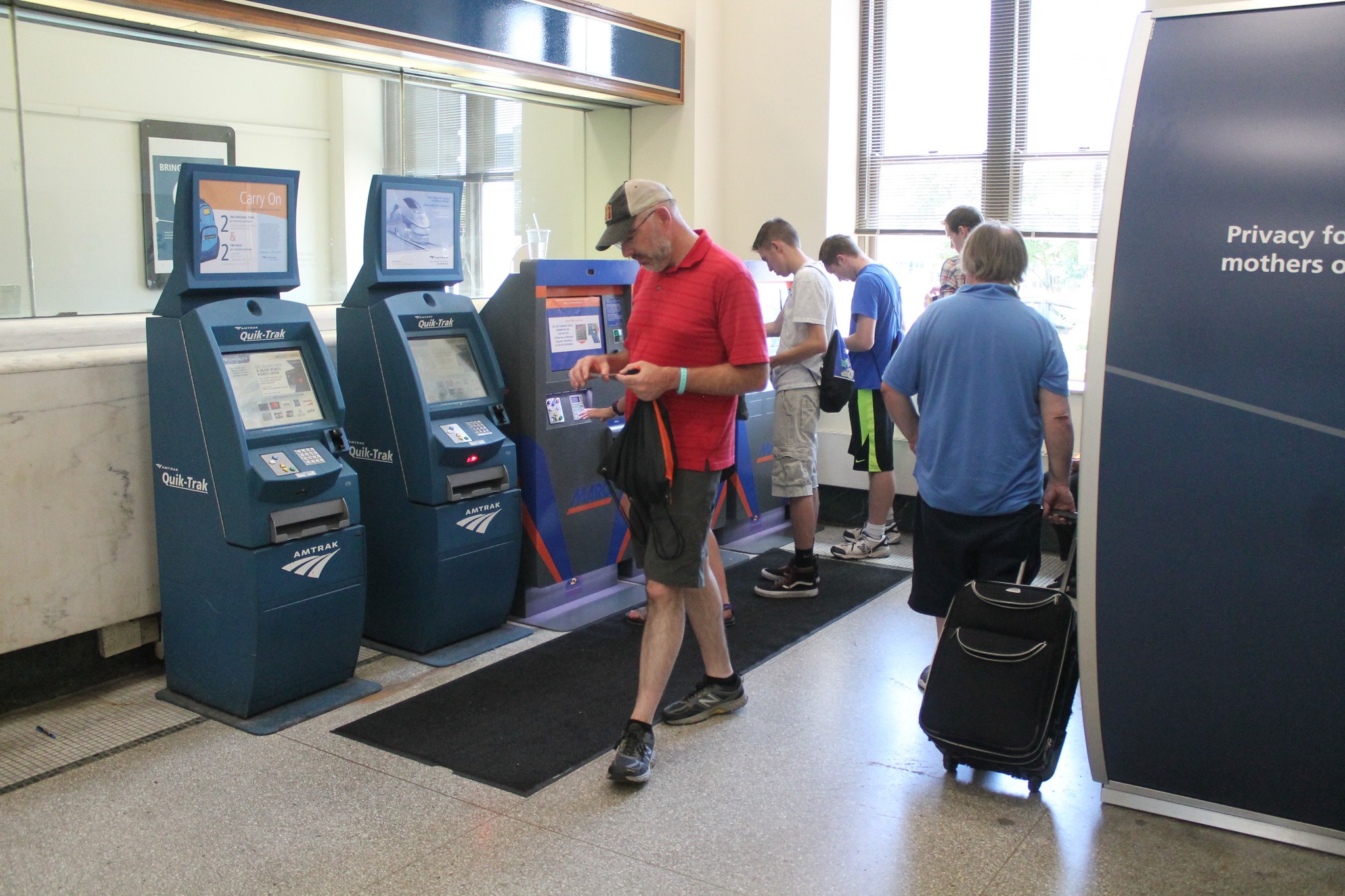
[819,329,855,413]
[596,397,675,499]
[891,330,905,356]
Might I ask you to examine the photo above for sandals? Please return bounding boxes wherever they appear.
[721,601,736,626]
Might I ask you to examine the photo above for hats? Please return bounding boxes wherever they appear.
[595,179,672,252]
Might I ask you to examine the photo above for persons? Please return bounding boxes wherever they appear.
[881,224,1079,690]
[925,206,981,306]
[816,234,904,562]
[750,218,835,596]
[567,179,769,786]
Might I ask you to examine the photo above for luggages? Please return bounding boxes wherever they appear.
[919,510,1078,791]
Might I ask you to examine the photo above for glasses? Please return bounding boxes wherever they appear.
[614,211,657,249]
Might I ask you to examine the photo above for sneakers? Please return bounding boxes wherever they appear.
[761,560,820,583]
[605,723,659,782]
[830,532,890,558]
[843,521,901,544]
[919,664,932,689]
[663,682,749,724]
[754,564,819,598]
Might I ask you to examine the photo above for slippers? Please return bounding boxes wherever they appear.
[625,606,648,625]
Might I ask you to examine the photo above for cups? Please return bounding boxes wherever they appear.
[525,230,551,260]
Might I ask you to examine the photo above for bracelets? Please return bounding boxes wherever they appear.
[611,401,625,416]
[676,367,687,395]
